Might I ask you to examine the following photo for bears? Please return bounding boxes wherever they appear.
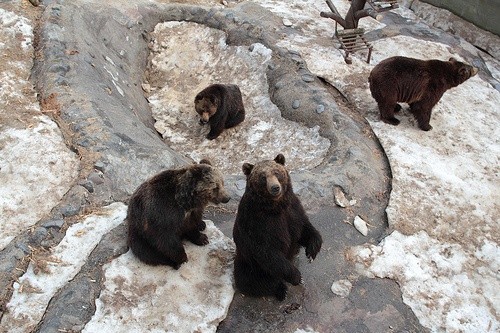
[194,83,245,140]
[232,153,323,302]
[368,56,478,132]
[126,159,231,270]
